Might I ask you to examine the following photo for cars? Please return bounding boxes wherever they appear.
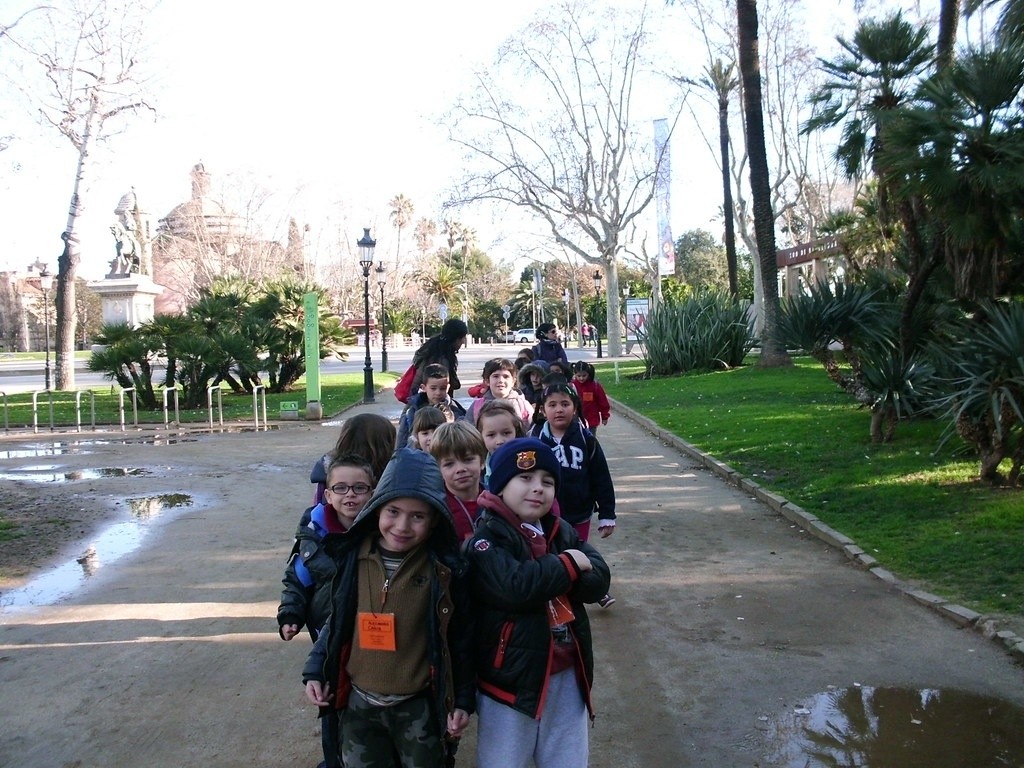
[501,331,518,342]
[515,328,536,343]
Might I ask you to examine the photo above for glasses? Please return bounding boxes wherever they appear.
[550,330,556,334]
[328,484,372,494]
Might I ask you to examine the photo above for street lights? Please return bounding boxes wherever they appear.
[375,261,390,373]
[36,266,55,388]
[355,227,377,403]
[592,269,604,358]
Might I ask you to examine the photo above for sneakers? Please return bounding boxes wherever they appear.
[597,593,616,610]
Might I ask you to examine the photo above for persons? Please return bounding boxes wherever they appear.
[459,438,611,768]
[409,319,467,400]
[588,323,596,347]
[302,449,477,768]
[530,323,567,365]
[526,382,615,609]
[277,452,377,768]
[582,322,589,346]
[310,349,610,539]
[573,326,578,340]
[123,210,137,256]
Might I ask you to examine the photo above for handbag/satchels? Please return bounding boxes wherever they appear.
[394,364,416,404]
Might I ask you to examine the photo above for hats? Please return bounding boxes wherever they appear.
[488,436,560,496]
[536,323,555,339]
